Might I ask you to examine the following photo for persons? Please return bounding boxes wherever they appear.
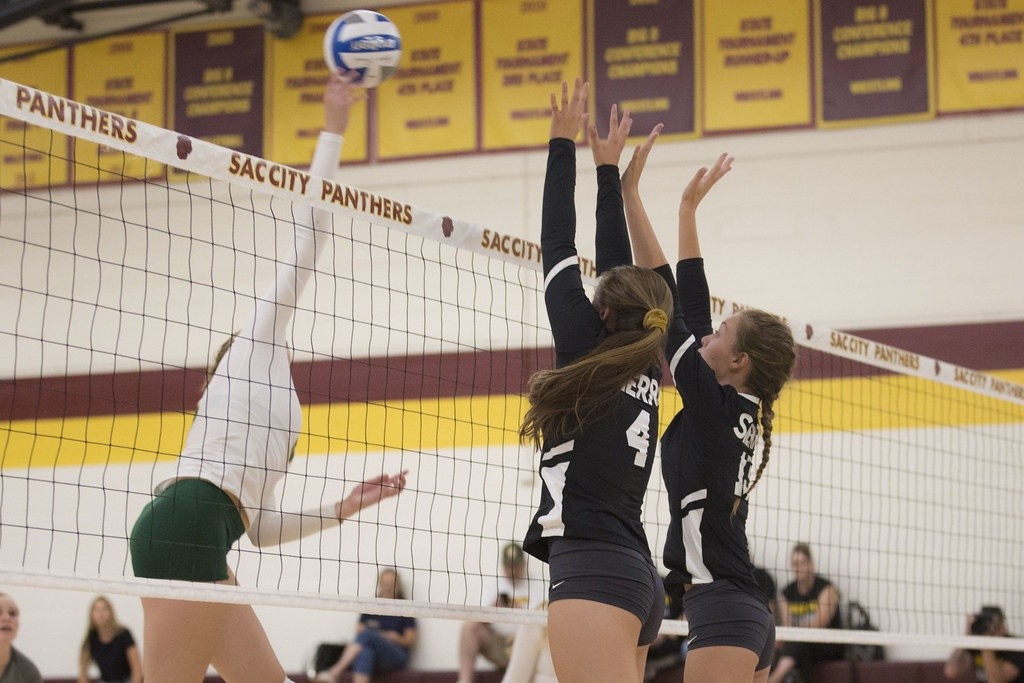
[130,67,409,683]
[621,123,795,683]
[78,595,142,683]
[458,543,548,683]
[317,568,416,683]
[769,544,844,683]
[519,78,674,683]
[945,606,1024,683]
[0,592,42,683]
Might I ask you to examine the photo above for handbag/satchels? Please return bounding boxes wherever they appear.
[843,601,885,661]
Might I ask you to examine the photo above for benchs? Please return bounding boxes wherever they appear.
[38,651,1024,683]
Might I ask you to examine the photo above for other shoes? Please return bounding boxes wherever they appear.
[315,672,337,683]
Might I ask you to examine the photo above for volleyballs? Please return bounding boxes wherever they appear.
[322,8,403,89]
